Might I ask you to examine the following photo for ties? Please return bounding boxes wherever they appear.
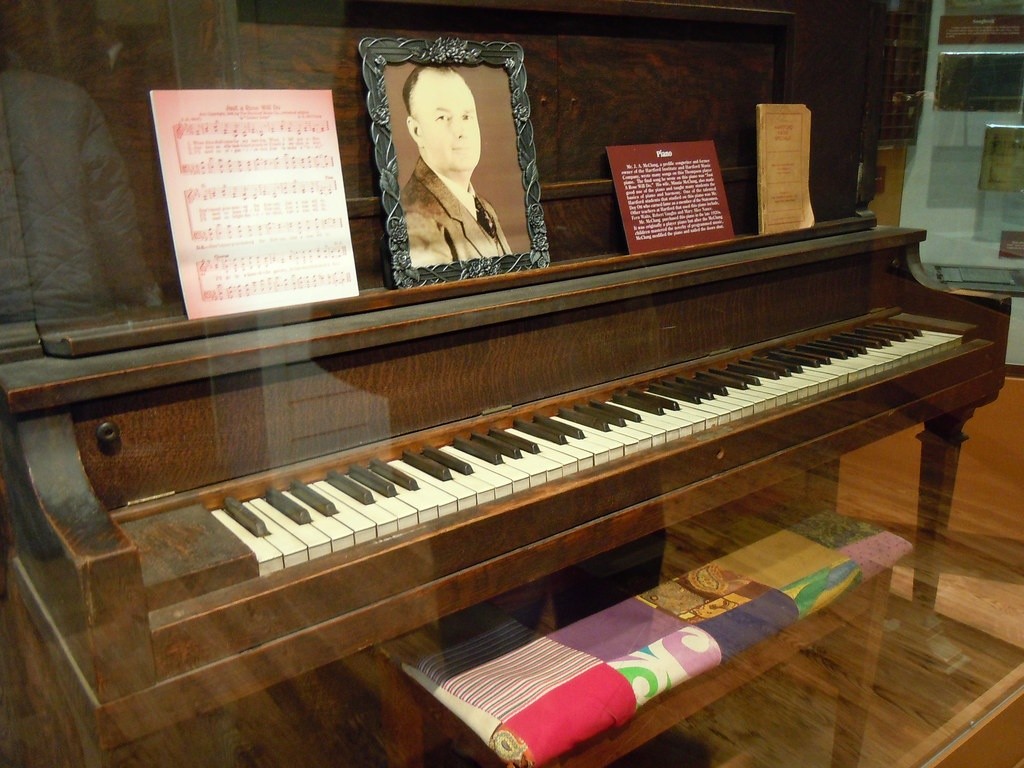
[475,196,494,238]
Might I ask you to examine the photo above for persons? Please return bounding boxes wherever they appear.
[0,16,164,326]
[395,62,510,269]
[312,215,665,662]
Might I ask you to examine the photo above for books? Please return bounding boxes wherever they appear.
[978,124,1024,194]
[757,104,814,236]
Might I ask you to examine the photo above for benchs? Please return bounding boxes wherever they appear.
[401,512,914,768]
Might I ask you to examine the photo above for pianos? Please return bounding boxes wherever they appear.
[0,1,1014,768]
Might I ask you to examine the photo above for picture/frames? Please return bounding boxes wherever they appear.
[357,35,551,291]
